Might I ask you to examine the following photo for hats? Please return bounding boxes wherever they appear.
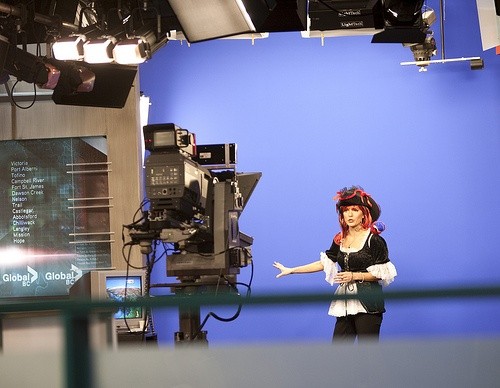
[335,185,381,223]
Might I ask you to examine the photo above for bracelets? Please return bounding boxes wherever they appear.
[350,272,353,282]
[359,272,364,283]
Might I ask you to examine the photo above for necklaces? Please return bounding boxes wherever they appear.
[347,234,361,248]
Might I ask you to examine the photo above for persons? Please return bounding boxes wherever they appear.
[272,185,397,348]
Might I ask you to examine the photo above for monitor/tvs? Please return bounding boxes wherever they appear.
[69,270,146,329]
